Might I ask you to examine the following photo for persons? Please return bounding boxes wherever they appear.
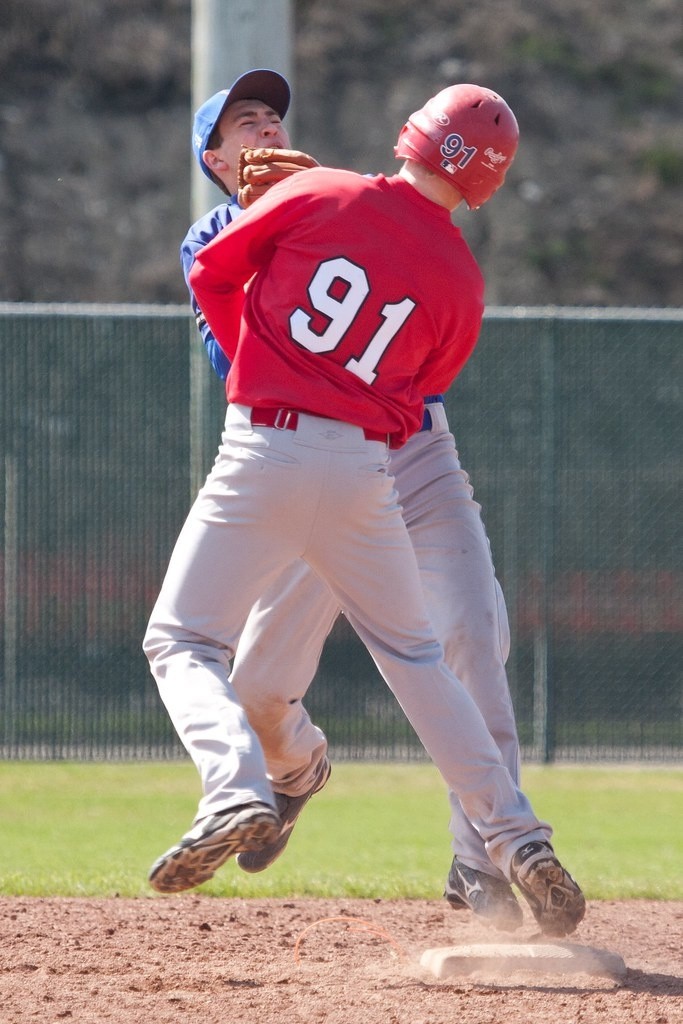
[141,80,586,937]
[180,69,524,930]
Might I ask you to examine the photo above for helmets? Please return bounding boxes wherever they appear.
[395,84,519,209]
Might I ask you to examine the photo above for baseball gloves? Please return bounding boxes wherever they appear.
[237,143,322,209]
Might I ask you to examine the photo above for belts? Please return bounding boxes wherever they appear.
[415,410,432,431]
[250,407,387,445]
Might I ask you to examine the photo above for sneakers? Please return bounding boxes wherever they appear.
[148,803,281,894]
[511,842,586,937]
[443,859,522,932]
[238,755,334,871]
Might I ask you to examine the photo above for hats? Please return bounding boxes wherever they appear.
[192,69,291,183]
